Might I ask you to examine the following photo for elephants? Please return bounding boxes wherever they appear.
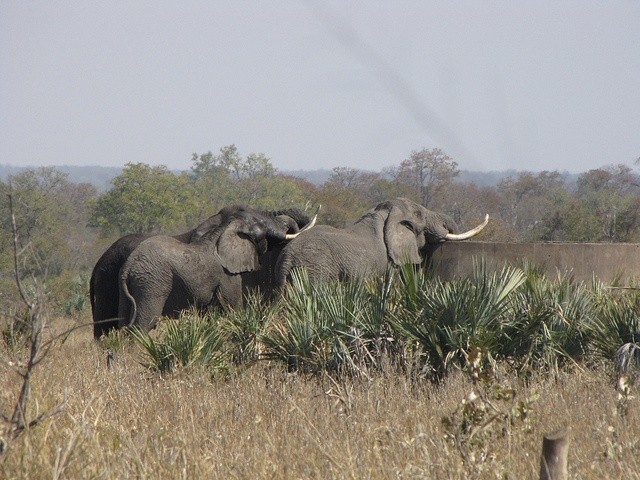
[273,196,490,291]
[117,212,319,333]
[89,197,323,340]
